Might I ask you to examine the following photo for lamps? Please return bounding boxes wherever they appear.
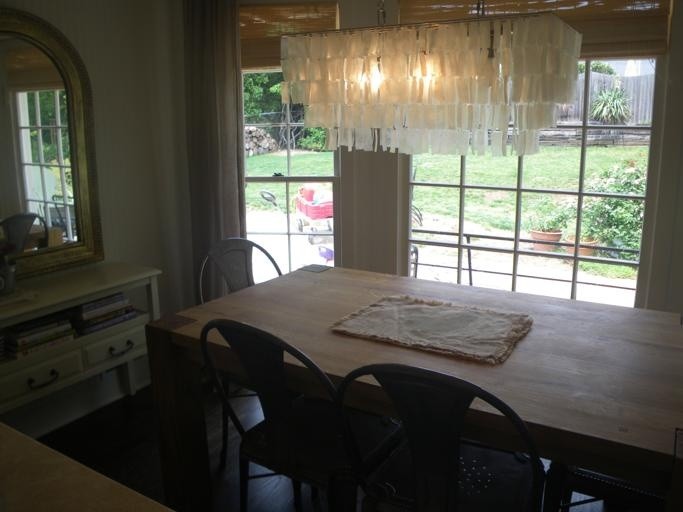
[265,1,584,162]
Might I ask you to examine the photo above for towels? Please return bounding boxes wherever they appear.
[325,292,532,367]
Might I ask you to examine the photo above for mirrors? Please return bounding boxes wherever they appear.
[0,7,105,288]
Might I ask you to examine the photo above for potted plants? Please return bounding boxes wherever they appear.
[531,197,598,261]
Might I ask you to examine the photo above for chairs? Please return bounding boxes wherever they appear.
[200,318,357,510]
[0,212,48,253]
[336,363,545,512]
[201,239,283,464]
[545,430,682,510]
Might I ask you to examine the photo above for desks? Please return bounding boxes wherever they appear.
[25,225,63,255]
[1,258,161,430]
[145,263,681,512]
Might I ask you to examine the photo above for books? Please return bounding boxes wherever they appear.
[0,291,138,362]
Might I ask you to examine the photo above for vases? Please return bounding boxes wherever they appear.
[0,265,17,295]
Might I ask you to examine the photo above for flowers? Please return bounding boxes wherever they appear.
[0,241,18,264]
